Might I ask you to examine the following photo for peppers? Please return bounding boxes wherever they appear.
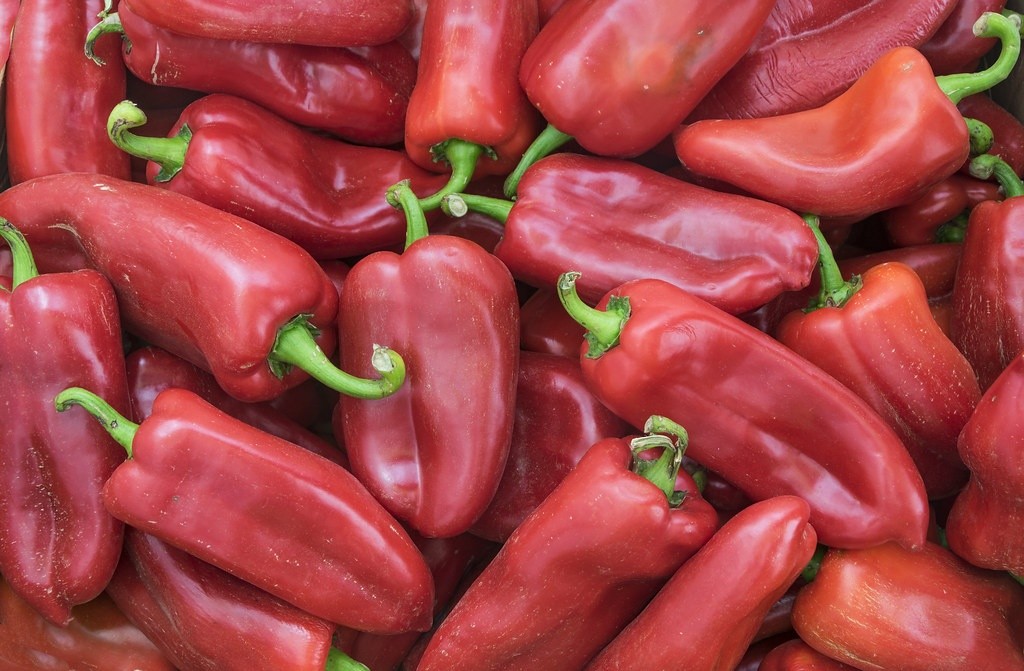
[0,0,1024,671]
